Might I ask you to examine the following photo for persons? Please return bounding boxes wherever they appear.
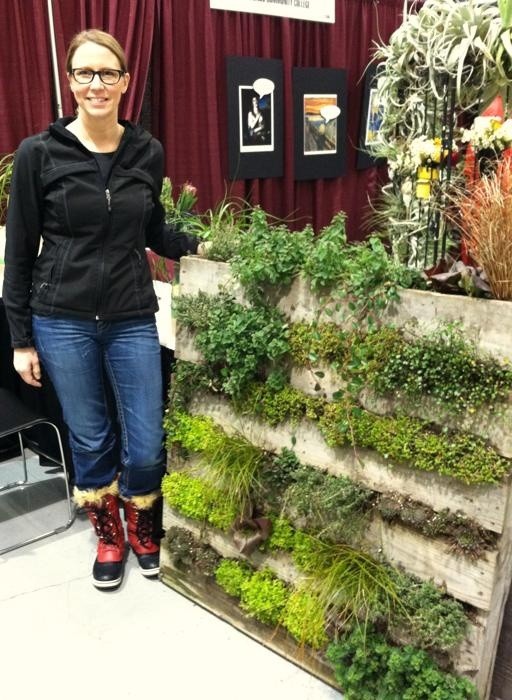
[247,97,269,142]
[2,27,215,591]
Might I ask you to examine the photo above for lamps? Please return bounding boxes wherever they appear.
[0,290,80,559]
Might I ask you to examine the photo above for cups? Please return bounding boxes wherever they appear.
[67,67,125,85]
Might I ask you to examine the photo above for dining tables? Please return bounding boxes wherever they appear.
[143,172,204,287]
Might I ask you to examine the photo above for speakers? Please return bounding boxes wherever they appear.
[70,481,128,589]
[118,491,164,581]
